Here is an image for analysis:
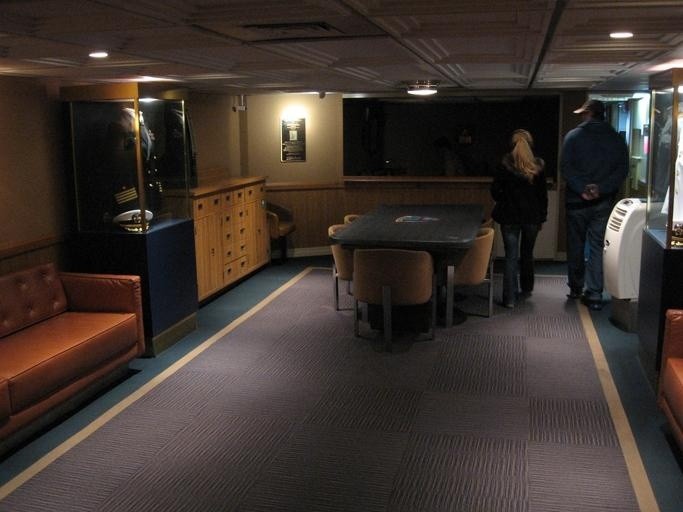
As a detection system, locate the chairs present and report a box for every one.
[265,196,296,264]
[324,200,499,354]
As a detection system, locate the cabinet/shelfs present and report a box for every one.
[156,171,274,309]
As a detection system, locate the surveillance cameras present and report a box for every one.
[232,105,245,112]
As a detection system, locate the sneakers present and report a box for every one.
[505,304,515,308]
[569,286,583,299]
[580,294,603,310]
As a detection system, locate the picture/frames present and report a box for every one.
[278,115,310,164]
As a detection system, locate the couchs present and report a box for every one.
[0,260,148,461]
[654,307,682,450]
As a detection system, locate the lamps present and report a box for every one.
[397,79,441,98]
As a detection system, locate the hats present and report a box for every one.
[572,97,606,116]
[110,209,154,233]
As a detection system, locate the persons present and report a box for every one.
[559,99,629,310]
[489,128,548,308]
